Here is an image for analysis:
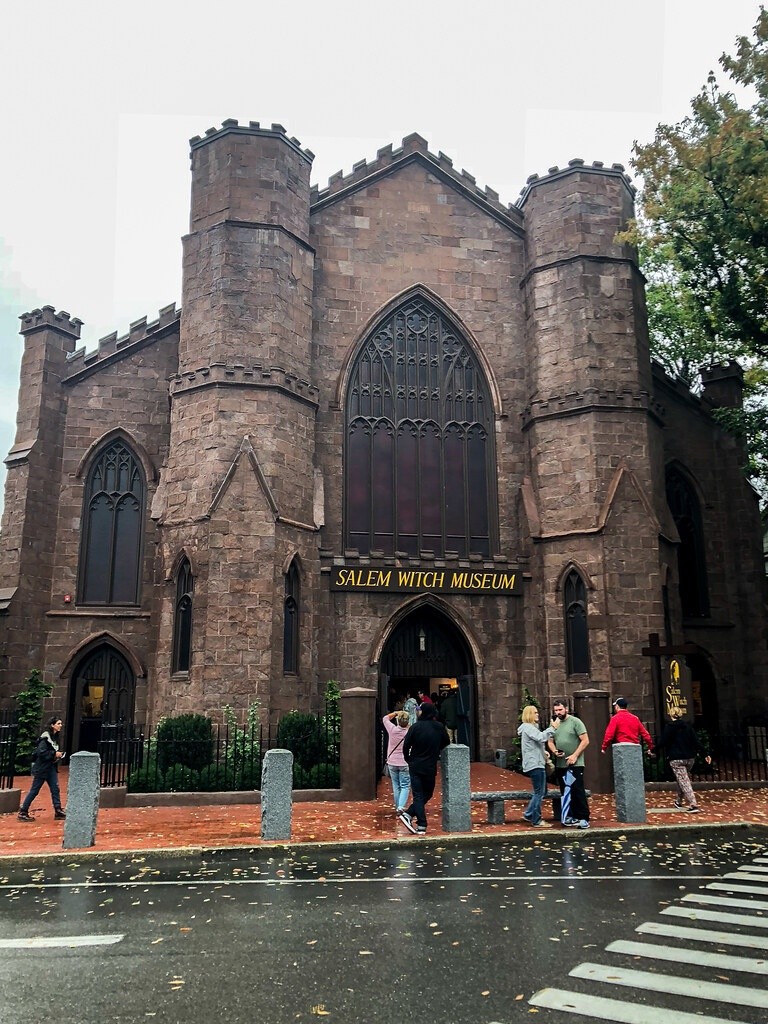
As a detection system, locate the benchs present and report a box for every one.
[471,788,591,825]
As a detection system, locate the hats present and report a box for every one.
[613,697,627,706]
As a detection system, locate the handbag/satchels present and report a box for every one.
[381,761,392,779]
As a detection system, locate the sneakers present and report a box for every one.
[399,812,417,835]
[397,809,403,816]
[415,825,427,836]
[533,820,555,828]
[686,807,700,814]
[522,814,533,822]
[674,801,682,808]
[55,812,66,820]
[16,814,35,822]
[561,817,573,827]
[575,819,591,829]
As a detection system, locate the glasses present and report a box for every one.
[535,711,538,714]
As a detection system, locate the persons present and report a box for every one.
[517,705,560,828]
[647,706,712,813]
[399,702,450,836]
[601,698,655,757]
[389,687,462,745]
[17,715,67,822]
[382,710,412,820]
[548,700,590,829]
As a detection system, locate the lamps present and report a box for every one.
[419,625,426,652]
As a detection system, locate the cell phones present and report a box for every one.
[552,715,558,721]
[558,749,564,755]
